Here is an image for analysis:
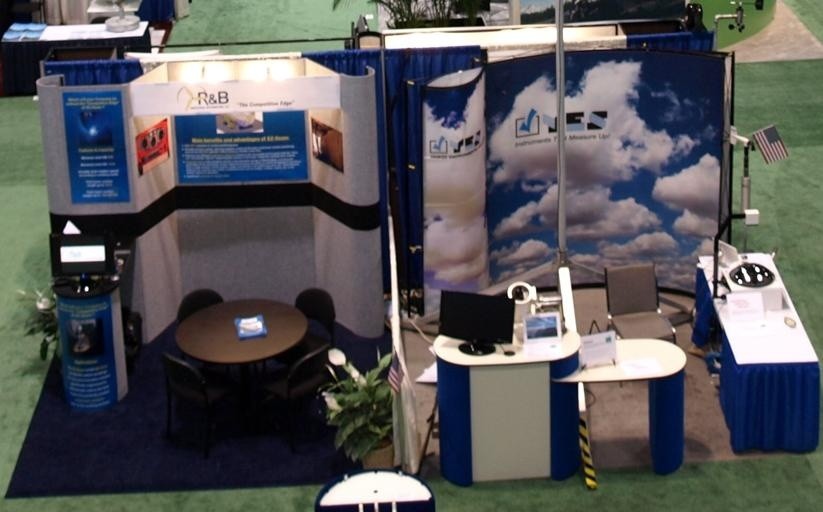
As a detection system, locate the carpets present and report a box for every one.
[402,282,772,471]
[5,331,393,500]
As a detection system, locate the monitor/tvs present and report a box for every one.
[49,233,114,293]
[438,290,516,357]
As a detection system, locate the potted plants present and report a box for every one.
[315,352,399,476]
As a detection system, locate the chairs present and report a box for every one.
[156,287,340,459]
[602,258,678,345]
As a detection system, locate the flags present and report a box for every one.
[385,352,407,394]
[751,125,790,165]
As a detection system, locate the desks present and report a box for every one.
[0,22,153,62]
[431,329,689,486]
[697,253,820,456]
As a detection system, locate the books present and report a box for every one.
[232,312,267,343]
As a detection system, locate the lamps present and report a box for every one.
[709,206,761,302]
